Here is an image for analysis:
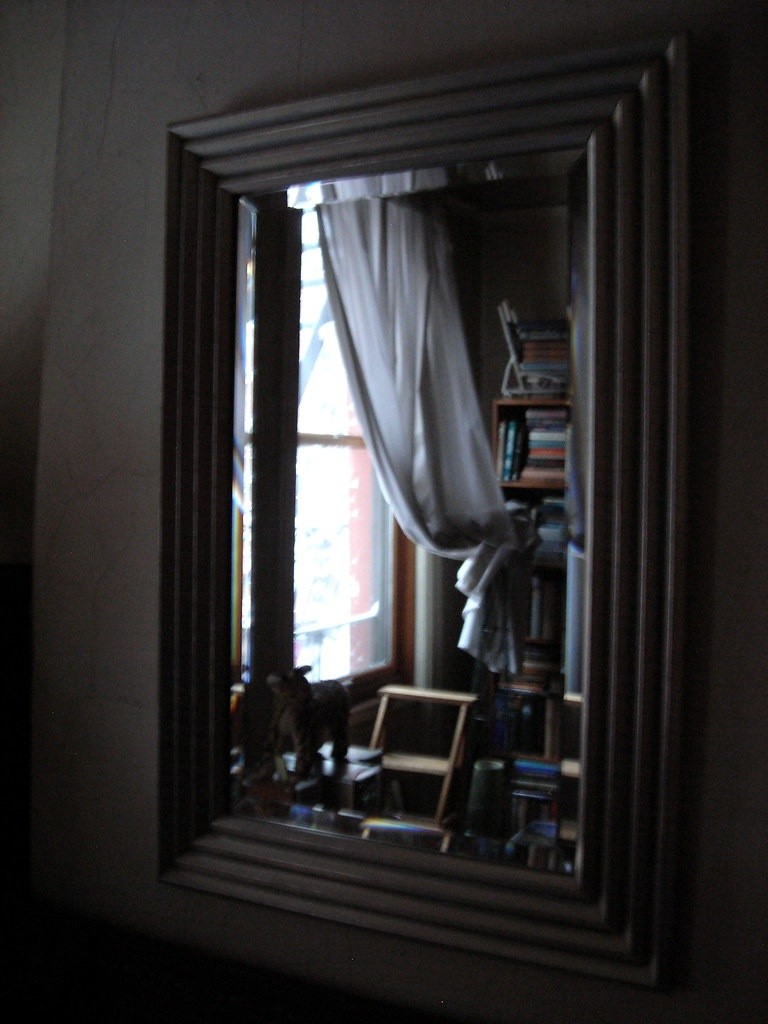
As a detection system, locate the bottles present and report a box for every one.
[290,804,336,832]
[527,566,563,645]
[522,638,560,687]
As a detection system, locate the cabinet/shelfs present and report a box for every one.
[473,304,581,763]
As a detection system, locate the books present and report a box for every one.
[518,319,569,371]
[491,647,550,750]
[528,575,555,641]
[495,409,568,483]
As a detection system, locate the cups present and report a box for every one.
[464,758,505,829]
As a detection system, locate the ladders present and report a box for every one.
[367,685,480,832]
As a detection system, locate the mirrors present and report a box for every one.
[152,35,730,998]
